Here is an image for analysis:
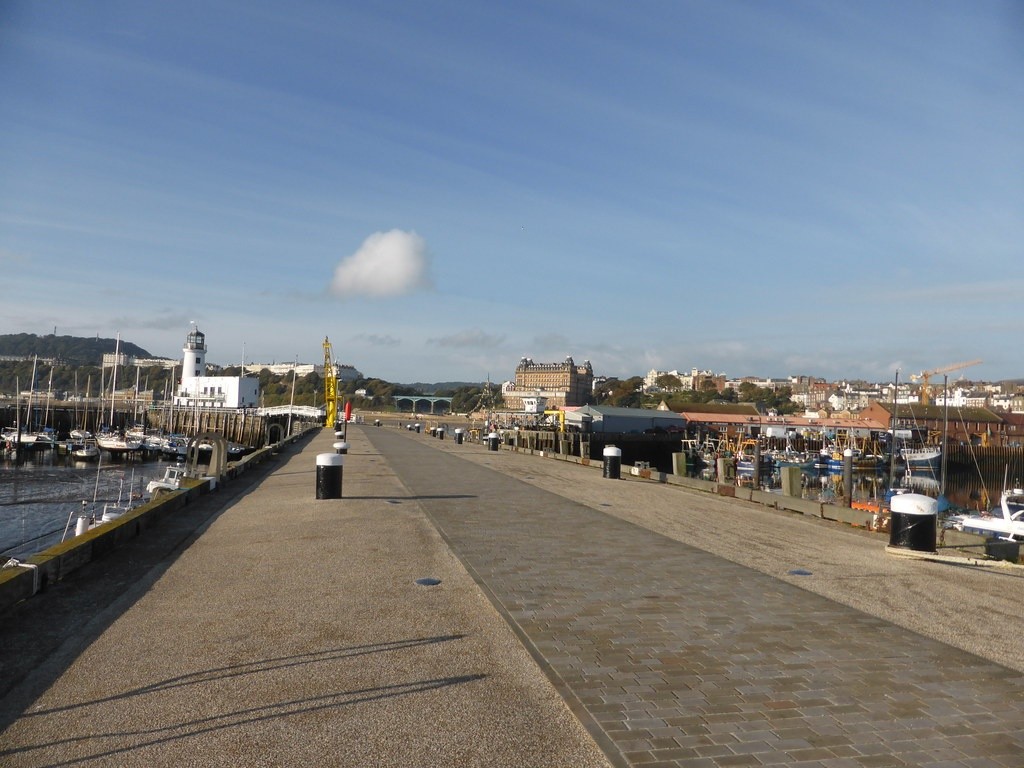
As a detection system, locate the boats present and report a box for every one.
[681,365,1024,544]
[0,331,257,533]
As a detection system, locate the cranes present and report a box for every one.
[910,360,982,406]
